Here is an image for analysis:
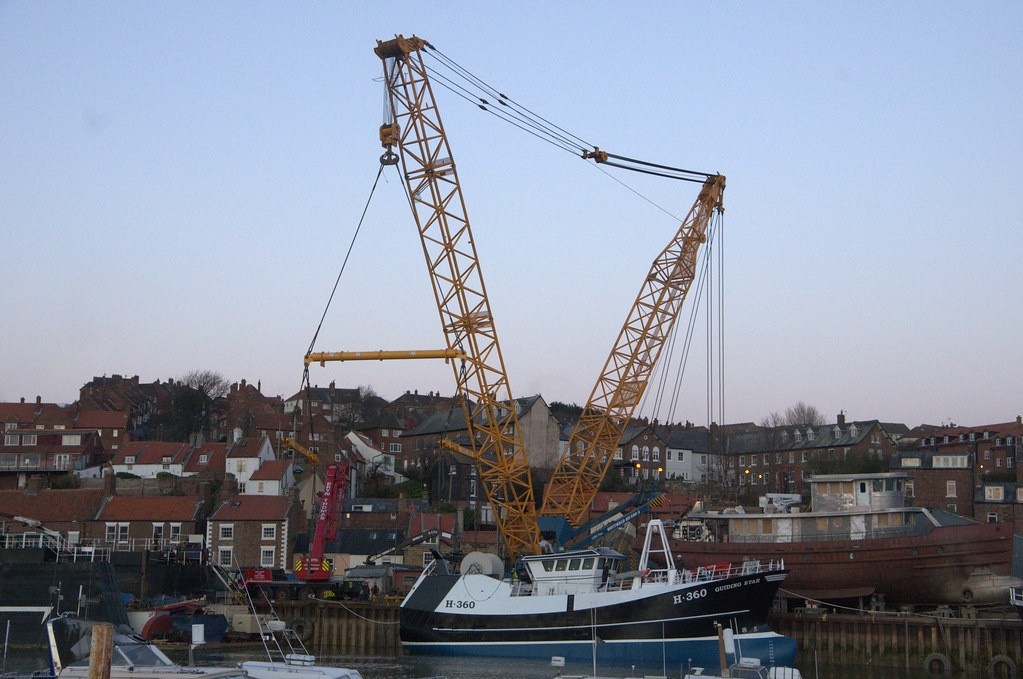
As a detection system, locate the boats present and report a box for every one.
[395,547,804,675]
[236,659,362,679]
[617,502,1016,608]
[28,637,248,679]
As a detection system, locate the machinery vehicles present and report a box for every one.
[294,460,347,581]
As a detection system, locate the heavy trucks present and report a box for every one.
[231,569,362,604]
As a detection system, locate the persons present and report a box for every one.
[153,530,159,551]
[675,555,684,571]
[597,563,609,592]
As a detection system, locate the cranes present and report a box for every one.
[282,35,726,563]
